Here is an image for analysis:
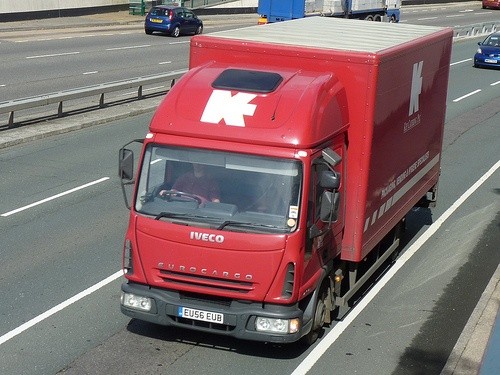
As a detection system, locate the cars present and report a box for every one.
[482,0,500,10]
[473,30,500,71]
[143,5,203,38]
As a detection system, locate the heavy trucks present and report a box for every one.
[256,0,403,27]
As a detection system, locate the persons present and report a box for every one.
[158,162,222,203]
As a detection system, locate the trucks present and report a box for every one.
[117,15,454,346]
